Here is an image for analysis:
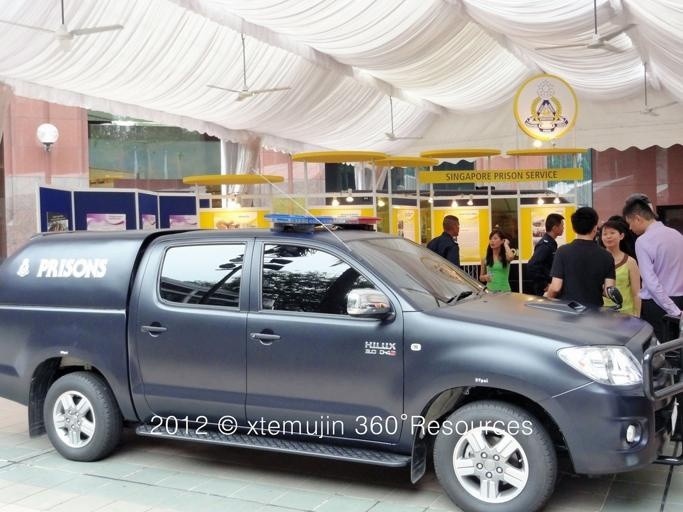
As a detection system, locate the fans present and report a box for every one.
[370,90,423,144]
[0,0,124,84]
[207,32,291,103]
[621,61,678,117]
[535,0,636,54]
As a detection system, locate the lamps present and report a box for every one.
[451,199,458,208]
[346,188,354,203]
[376,196,385,207]
[331,192,339,208]
[536,194,545,204]
[552,192,561,204]
[36,122,60,153]
[466,193,474,206]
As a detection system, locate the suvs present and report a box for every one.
[0,214,676,512]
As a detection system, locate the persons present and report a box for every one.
[525,194,683,443]
[479,230,516,293]
[425,214,460,268]
